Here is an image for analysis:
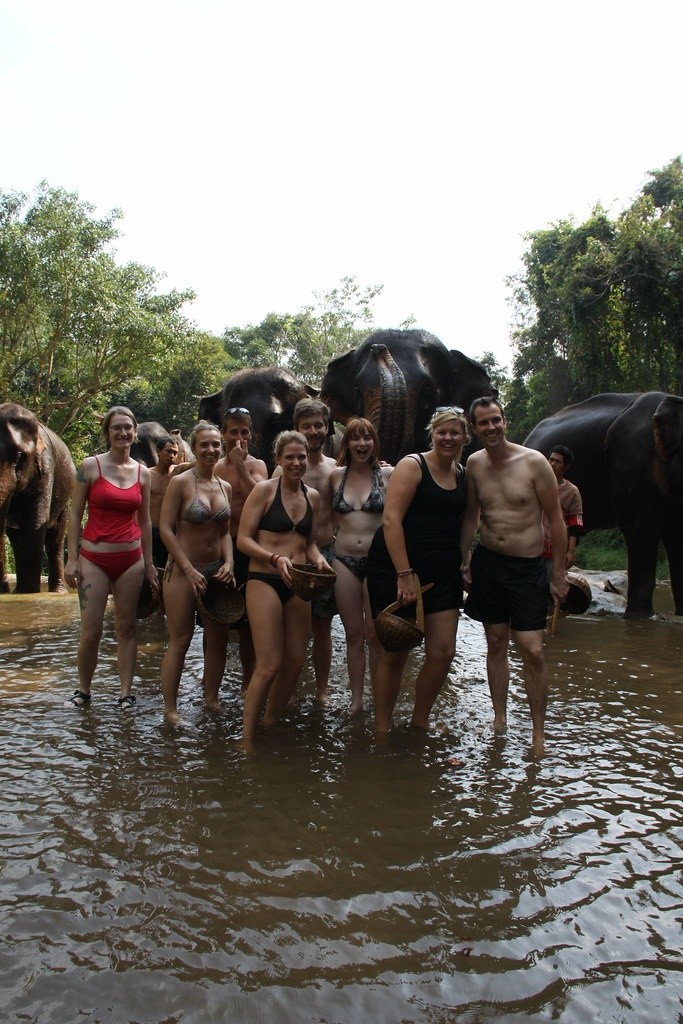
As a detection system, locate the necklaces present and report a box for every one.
[195,474,218,492]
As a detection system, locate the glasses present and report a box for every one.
[223,408,250,419]
[433,405,464,417]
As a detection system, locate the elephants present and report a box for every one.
[319,329,500,464]
[0,402,78,594]
[130,422,196,469]
[198,370,345,478]
[525,391,683,622]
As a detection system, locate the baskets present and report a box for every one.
[546,577,592,635]
[195,576,245,626]
[373,573,434,652]
[135,579,161,619]
[287,563,337,601]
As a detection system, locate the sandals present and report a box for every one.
[64,690,92,709]
[118,696,138,713]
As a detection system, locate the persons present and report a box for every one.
[541,445,583,635]
[160,420,236,718]
[460,396,571,757]
[272,398,392,700]
[236,430,336,738]
[64,406,160,710]
[148,436,178,619]
[366,406,466,733]
[196,407,268,698]
[329,418,395,710]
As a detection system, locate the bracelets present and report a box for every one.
[270,554,278,565]
[273,555,281,568]
[397,569,413,577]
